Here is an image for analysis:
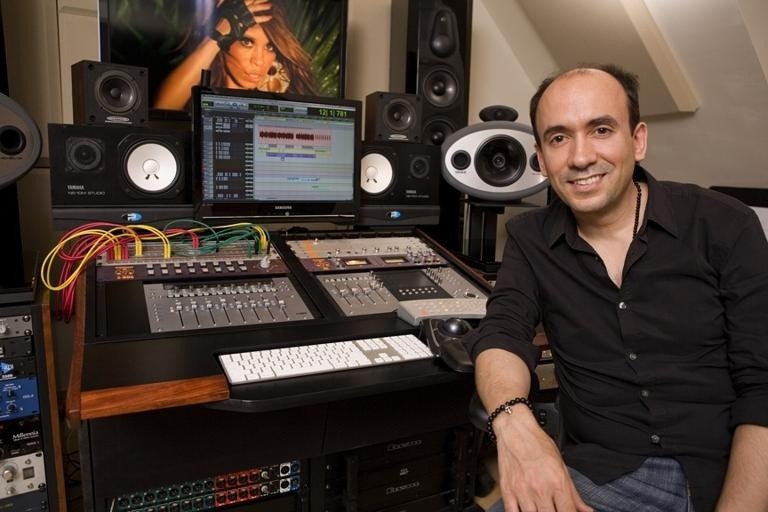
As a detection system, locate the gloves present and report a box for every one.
[210,0,257,51]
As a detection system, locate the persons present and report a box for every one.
[153,0,321,111]
[470,62,768,512]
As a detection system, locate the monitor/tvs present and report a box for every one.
[189,84,364,226]
[96,2,349,124]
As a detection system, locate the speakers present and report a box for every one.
[71,58,149,126]
[2,94,44,185]
[365,90,425,146]
[389,0,473,254]
[359,142,443,227]
[441,103,550,207]
[46,120,201,212]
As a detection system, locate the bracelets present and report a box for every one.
[485,397,534,442]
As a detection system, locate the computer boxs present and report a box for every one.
[0,247,70,512]
[341,434,474,511]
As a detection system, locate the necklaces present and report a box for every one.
[631,177,643,239]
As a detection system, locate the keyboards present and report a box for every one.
[215,333,434,387]
